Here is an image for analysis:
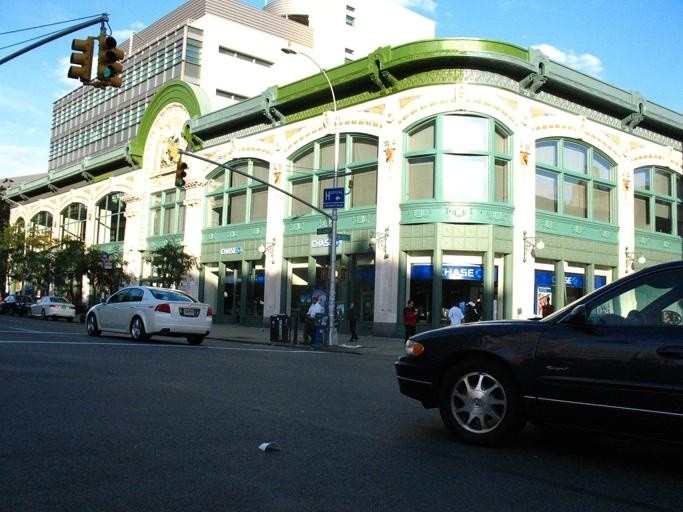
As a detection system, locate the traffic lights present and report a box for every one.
[111,47,125,88]
[175,161,187,187]
[67,38,94,82]
[100,36,117,81]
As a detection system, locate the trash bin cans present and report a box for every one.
[312,313,327,345]
[270,314,290,342]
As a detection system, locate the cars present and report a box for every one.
[86,285,214,346]
[0,295,35,317]
[27,295,77,322]
[392,259,683,448]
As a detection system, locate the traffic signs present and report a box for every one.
[281,46,367,351]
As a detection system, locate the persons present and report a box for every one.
[346,301,359,343]
[14,291,20,295]
[403,299,418,344]
[64,290,72,302]
[447,299,481,327]
[304,295,324,350]
